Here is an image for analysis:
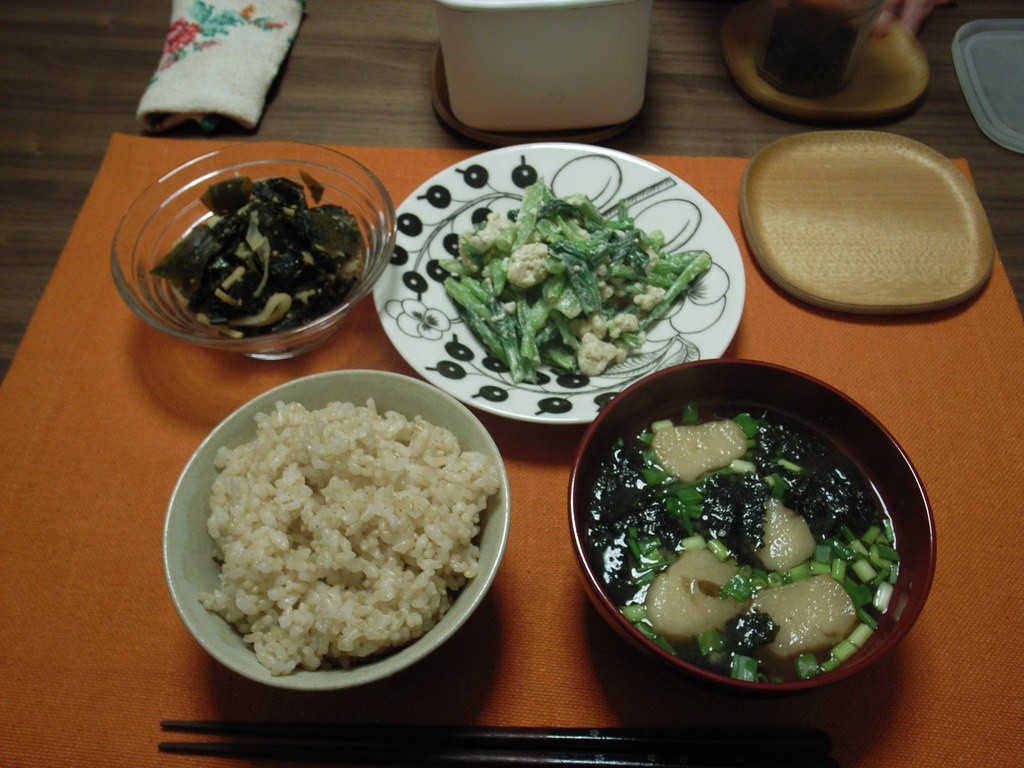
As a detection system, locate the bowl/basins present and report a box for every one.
[565,360,938,697]
[435,0,648,130]
[107,139,398,361]
[164,370,512,689]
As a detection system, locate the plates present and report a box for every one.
[372,142,746,424]
[431,42,638,145]
[717,0,928,121]
[737,131,995,314]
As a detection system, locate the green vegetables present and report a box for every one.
[442,181,710,382]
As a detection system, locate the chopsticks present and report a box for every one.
[158,719,836,768]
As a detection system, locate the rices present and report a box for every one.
[194,398,502,679]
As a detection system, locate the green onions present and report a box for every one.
[606,401,901,683]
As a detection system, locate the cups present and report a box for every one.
[753,0,885,99]
